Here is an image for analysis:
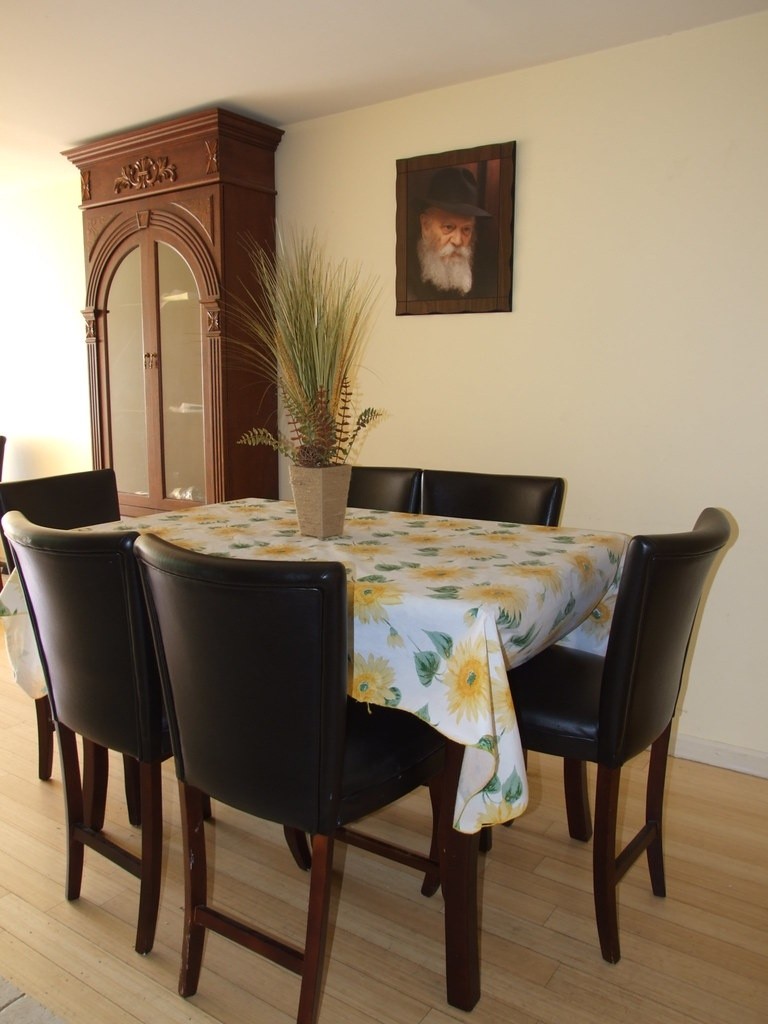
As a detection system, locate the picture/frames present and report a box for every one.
[392,136,518,316]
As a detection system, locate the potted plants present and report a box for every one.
[216,242,383,539]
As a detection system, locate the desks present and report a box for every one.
[0,497,633,1015]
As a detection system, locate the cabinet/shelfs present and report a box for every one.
[61,108,286,517]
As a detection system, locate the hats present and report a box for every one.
[412,167,493,217]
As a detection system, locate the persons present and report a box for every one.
[406,166,497,300]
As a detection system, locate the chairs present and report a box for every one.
[418,508,734,965]
[0,508,176,952]
[421,468,567,527]
[1,470,122,782]
[348,465,417,514]
[135,533,482,1024]
